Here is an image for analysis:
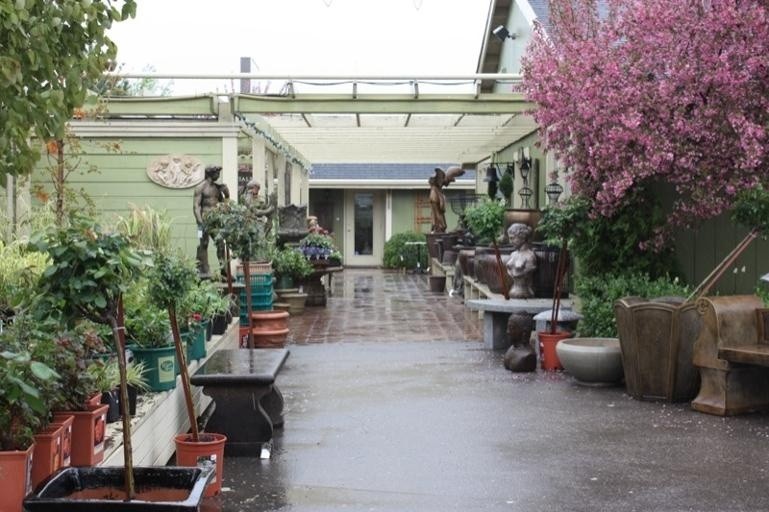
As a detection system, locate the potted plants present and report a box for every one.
[268,246,315,287]
[536,196,589,375]
[0,193,233,512]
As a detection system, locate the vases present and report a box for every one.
[475,243,515,294]
[423,233,475,292]
[557,333,627,389]
[246,289,308,347]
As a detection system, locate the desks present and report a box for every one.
[190,347,290,457]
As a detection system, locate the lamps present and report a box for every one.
[483,162,503,199]
[519,158,533,180]
[492,24,516,41]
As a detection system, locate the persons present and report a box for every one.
[505,221,537,298]
[243,178,274,240]
[192,164,238,285]
